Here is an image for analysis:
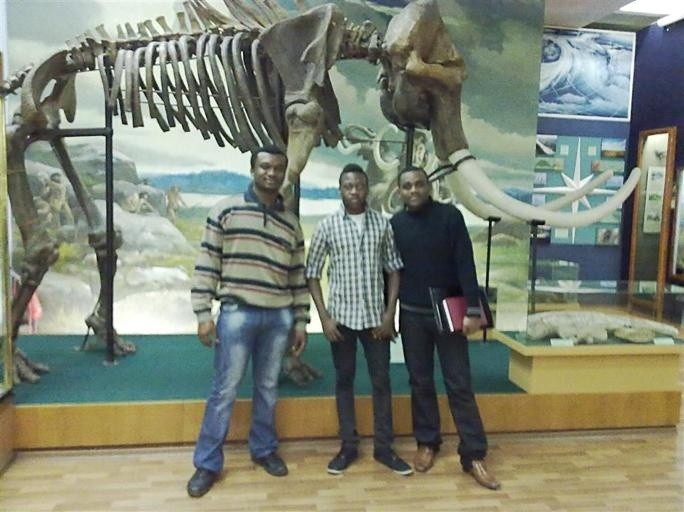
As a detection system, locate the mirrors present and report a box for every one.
[629,124,676,316]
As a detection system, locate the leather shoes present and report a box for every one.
[413,445,437,472]
[186,467,216,498]
[461,459,501,490]
[250,450,289,476]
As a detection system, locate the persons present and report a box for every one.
[539,31,632,105]
[185,144,311,498]
[381,167,503,491]
[304,162,417,480]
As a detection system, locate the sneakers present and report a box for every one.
[327,445,361,475]
[373,445,414,476]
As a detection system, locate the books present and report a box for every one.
[426,283,494,334]
[435,302,449,334]
[443,294,489,334]
